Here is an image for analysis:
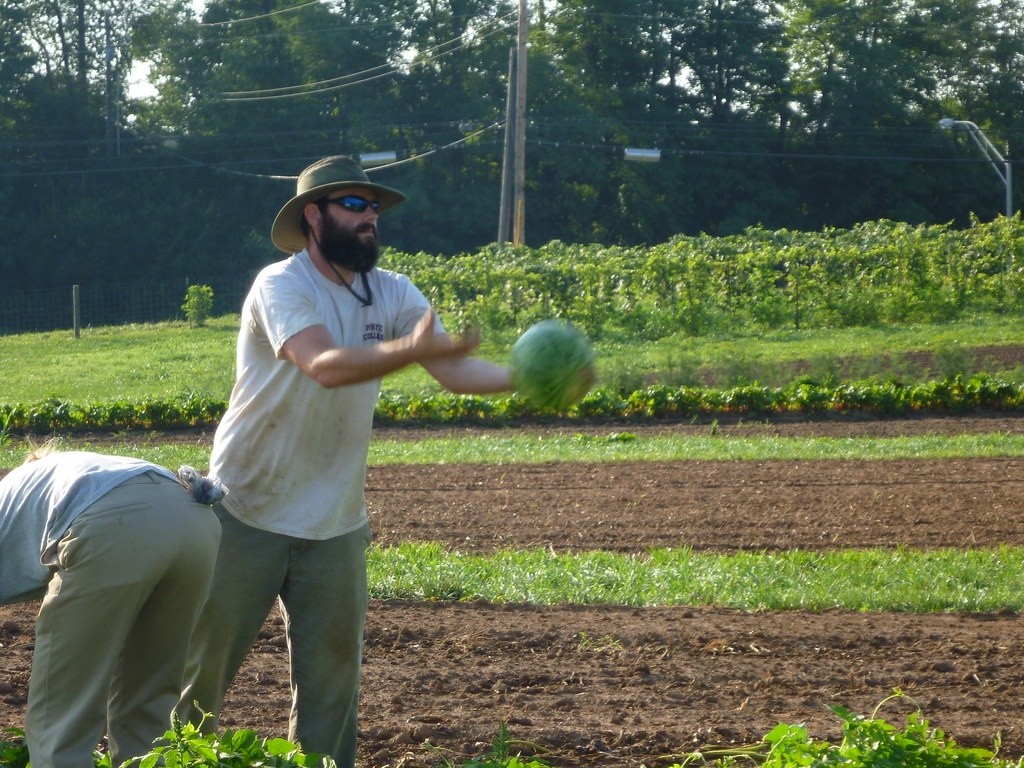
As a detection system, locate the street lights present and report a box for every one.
[939,116,1012,218]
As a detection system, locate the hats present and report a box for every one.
[273,154,407,254]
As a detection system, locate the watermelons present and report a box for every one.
[509,319,595,410]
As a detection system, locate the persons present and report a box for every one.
[210,156,519,768]
[0,450,223,768]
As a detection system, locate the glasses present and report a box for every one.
[325,196,382,215]
